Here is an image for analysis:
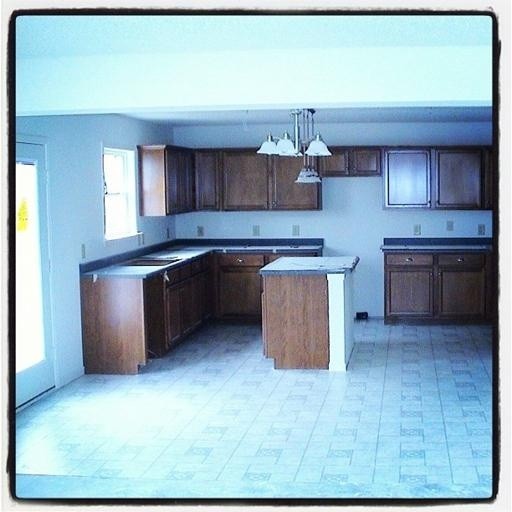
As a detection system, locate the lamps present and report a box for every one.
[256,108,332,184]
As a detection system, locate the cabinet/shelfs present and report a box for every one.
[320,144,383,176]
[383,144,492,209]
[137,144,194,217]
[160,252,214,356]
[383,249,487,325]
[193,148,221,210]
[222,147,321,211]
[266,252,317,265]
[215,254,265,320]
